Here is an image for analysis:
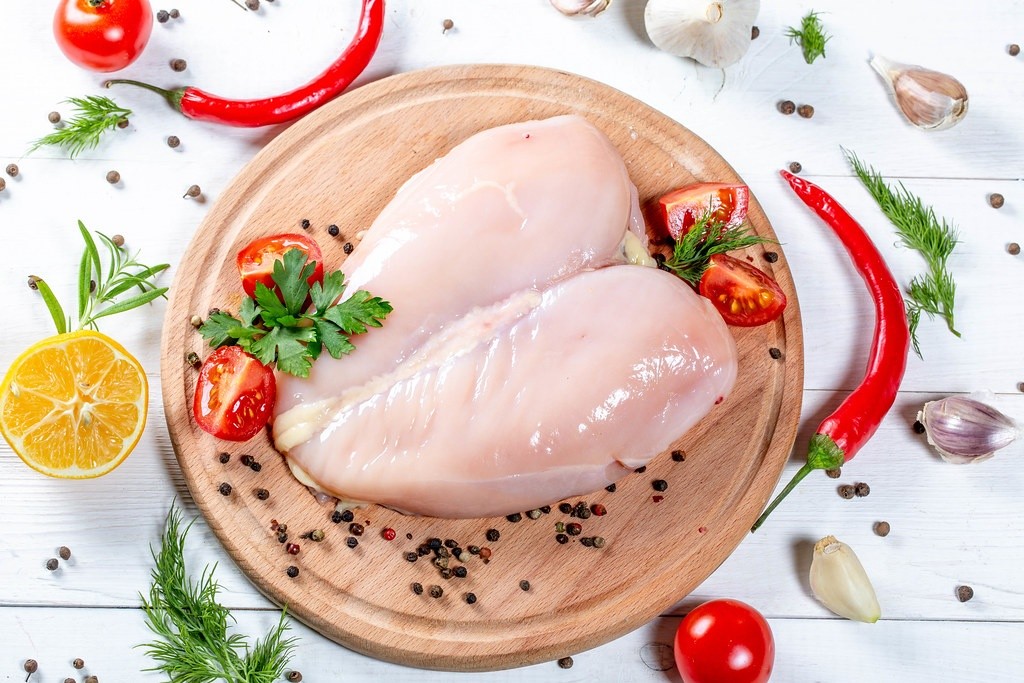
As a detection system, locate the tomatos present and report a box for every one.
[673,599,775,683]
[55,0,155,70]
[194,233,322,440]
[660,184,785,327]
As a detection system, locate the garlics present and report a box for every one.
[553,0,760,64]
[869,55,968,133]
[809,536,881,623]
[918,397,1020,460]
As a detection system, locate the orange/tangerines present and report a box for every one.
[0,331,147,477]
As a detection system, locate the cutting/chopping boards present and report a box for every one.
[160,62,806,671]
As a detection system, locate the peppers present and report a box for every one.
[106,1,386,128]
[751,170,909,538]
